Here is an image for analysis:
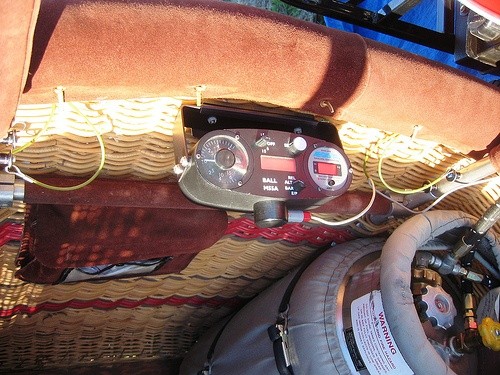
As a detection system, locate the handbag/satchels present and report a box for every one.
[15,203,228,285]
